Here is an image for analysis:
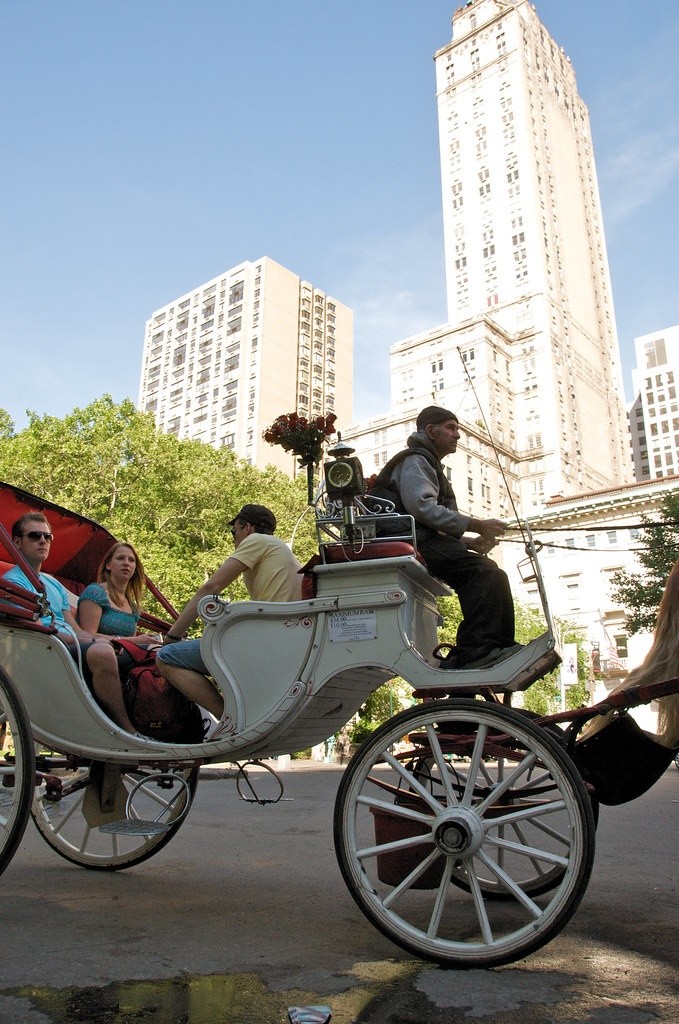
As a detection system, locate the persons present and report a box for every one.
[76,541,164,644]
[0,509,163,743]
[156,503,304,720]
[364,405,515,669]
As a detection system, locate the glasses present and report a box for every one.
[231,522,253,539]
[22,531,54,542]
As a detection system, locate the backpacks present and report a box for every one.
[111,638,212,743]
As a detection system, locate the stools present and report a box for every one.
[321,542,452,599]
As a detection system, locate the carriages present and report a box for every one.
[0,483,679,969]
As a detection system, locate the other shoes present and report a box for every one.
[440,644,522,670]
[134,731,165,743]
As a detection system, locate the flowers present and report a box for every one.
[262,412,338,503]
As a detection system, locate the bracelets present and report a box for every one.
[166,633,182,641]
[93,637,95,643]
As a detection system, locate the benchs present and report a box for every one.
[0,561,88,647]
[199,555,315,736]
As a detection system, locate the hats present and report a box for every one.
[417,406,459,432]
[226,504,276,532]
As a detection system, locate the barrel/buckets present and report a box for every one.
[368,792,461,890]
